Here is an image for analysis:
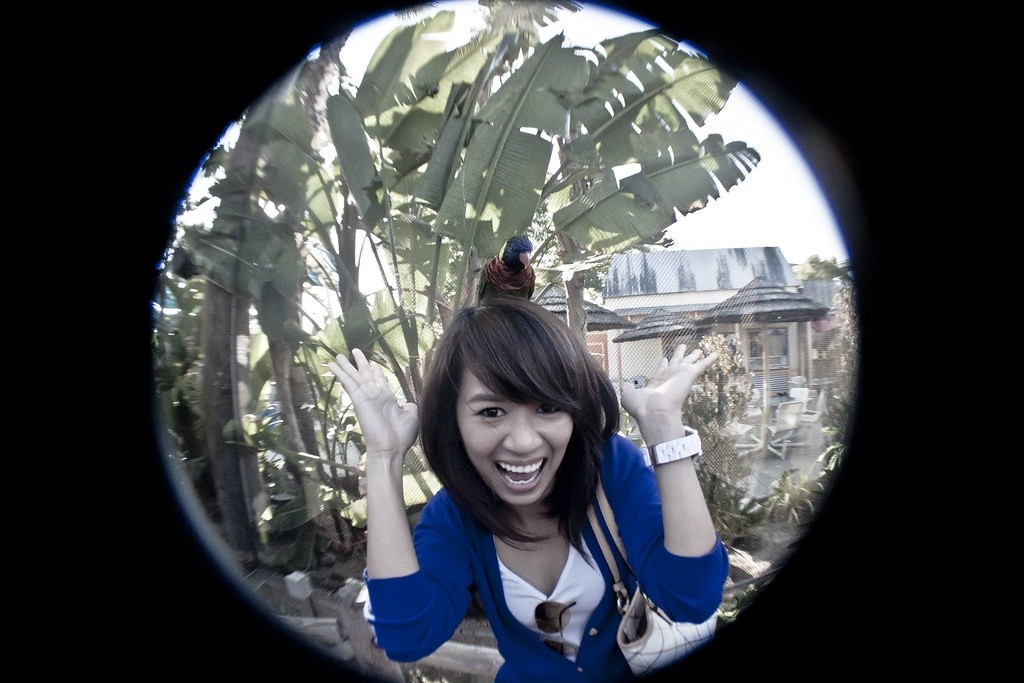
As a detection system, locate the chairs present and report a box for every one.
[718,375,827,460]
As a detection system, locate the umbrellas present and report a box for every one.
[526,275,830,459]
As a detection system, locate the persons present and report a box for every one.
[325,293,729,683]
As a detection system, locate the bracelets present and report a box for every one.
[647,424,705,466]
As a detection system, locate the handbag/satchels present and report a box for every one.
[586,475,726,676]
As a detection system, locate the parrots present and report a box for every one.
[475,235,537,307]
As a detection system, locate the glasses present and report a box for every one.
[534,600,578,658]
